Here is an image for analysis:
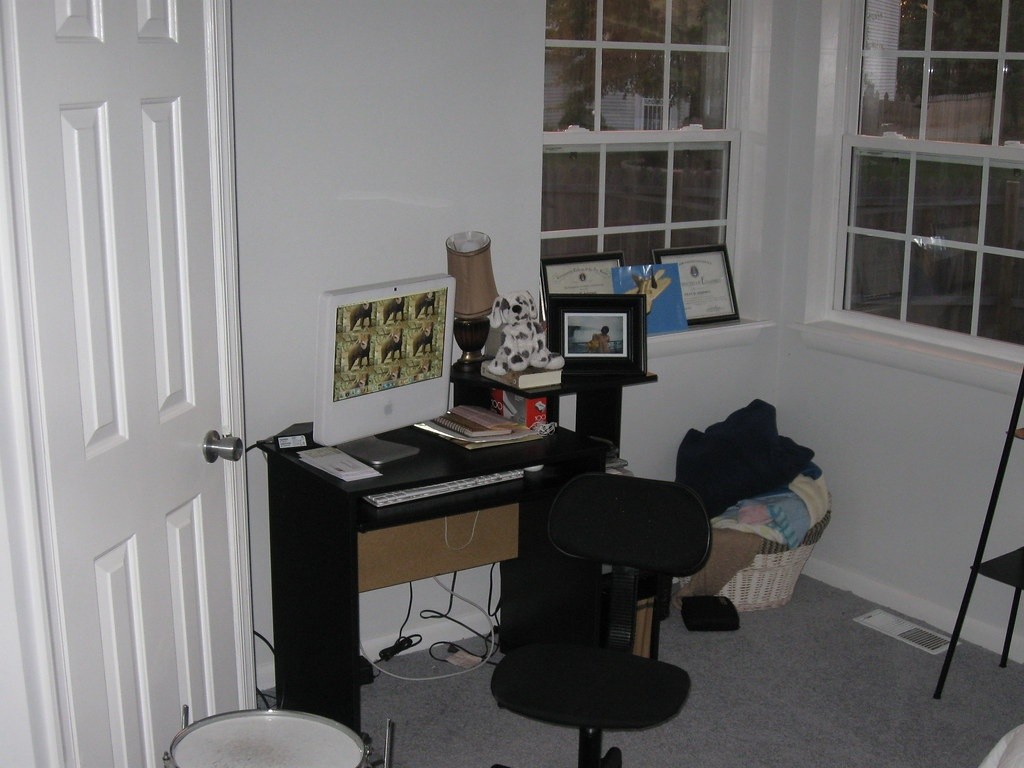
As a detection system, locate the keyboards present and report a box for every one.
[363,470,524,507]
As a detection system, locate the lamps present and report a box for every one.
[445,230,499,374]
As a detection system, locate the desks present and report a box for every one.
[450,356,657,457]
[254,427,609,747]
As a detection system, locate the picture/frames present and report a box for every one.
[652,244,741,328]
[540,250,626,316]
[546,293,648,377]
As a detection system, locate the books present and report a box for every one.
[414,405,543,451]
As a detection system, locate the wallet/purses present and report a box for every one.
[681,595,740,631]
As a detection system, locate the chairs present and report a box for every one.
[491,471,713,768]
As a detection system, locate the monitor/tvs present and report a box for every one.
[313,273,456,464]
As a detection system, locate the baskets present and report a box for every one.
[673,490,833,613]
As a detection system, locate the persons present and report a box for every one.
[587,326,611,353]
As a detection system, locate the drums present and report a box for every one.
[163,705,368,768]
